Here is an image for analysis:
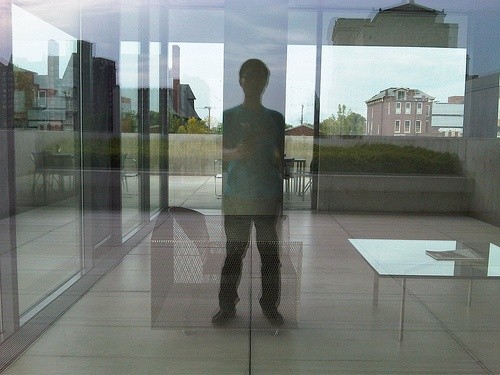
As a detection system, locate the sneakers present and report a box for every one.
[212,299,237,323]
[258,297,284,326]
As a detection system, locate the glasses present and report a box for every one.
[242,74,267,82]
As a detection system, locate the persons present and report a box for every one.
[209,58,285,328]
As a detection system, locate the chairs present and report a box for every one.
[300,160,311,198]
[123,154,128,192]
[42,155,74,199]
[29,152,59,194]
[284,158,293,201]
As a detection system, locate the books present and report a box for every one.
[426,247,484,261]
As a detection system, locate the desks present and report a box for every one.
[295,159,306,201]
[348,239,500,343]
